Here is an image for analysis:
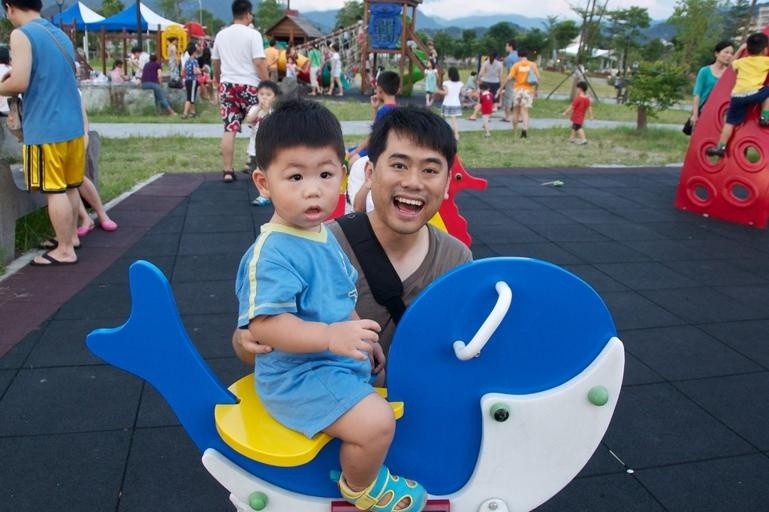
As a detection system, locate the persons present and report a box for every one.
[707,33,769,155]
[69,62,121,236]
[345,70,412,174]
[111,37,214,118]
[244,81,290,209]
[265,38,344,97]
[690,41,734,132]
[232,99,429,511]
[339,14,366,50]
[562,82,594,145]
[440,39,541,140]
[0,0,117,266]
[0,47,24,118]
[423,58,439,109]
[233,106,475,368]
[209,0,272,185]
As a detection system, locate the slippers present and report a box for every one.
[100,218,118,232]
[30,251,80,267]
[76,223,97,237]
[38,236,83,250]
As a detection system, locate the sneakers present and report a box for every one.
[705,146,726,158]
[337,464,428,512]
[758,116,769,129]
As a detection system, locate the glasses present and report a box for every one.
[249,10,253,17]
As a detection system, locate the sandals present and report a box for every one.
[222,168,237,183]
[250,195,272,207]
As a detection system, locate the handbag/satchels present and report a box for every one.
[6,95,24,144]
[317,65,322,77]
[527,68,540,85]
[682,115,692,136]
